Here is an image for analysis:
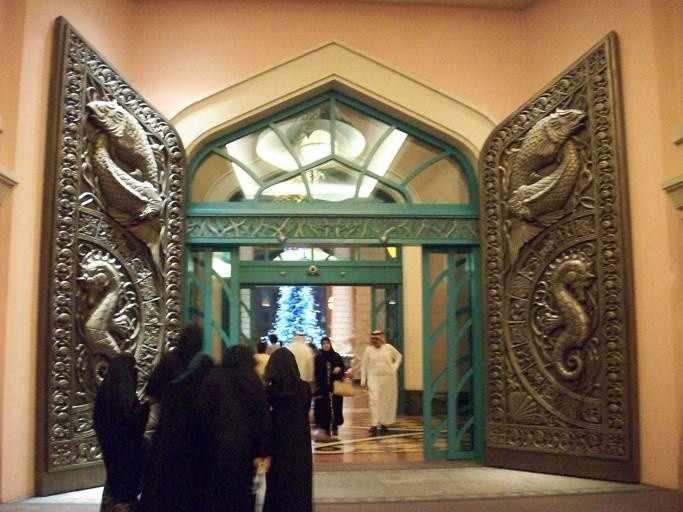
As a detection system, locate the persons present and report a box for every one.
[360,330,402,434]
[313,336,345,437]
[91,321,321,512]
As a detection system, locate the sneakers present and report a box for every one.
[369,425,387,434]
[324,425,338,436]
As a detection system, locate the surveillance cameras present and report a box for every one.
[309,266,317,273]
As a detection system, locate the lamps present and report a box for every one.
[255,118,366,173]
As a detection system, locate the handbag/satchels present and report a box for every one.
[334,380,355,397]
